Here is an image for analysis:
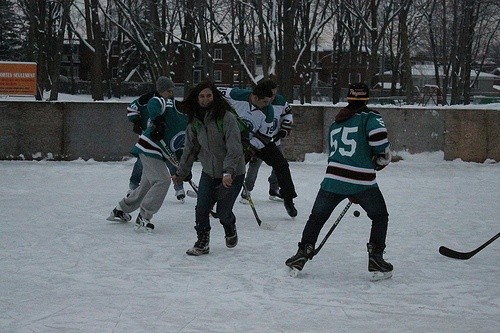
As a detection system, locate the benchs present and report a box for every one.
[460,92,500,105]
[369,96,408,105]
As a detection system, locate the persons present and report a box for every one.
[167,81,246,256]
[106,97,201,233]
[216,85,298,220]
[228,80,292,205]
[285,82,394,282]
[126,76,186,204]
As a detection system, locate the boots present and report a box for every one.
[223,224,238,248]
[367,242,393,280]
[285,242,311,277]
[186,226,210,255]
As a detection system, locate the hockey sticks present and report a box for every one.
[242,183,278,231]
[439,232,500,260]
[159,139,221,219]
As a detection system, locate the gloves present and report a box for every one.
[272,130,286,142]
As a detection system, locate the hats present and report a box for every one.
[347,82,370,100]
[156,76,174,92]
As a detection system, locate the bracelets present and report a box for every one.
[223,173,232,176]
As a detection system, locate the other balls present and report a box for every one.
[354,211,360,218]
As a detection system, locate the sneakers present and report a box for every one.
[135,213,154,230]
[241,187,251,200]
[107,208,131,222]
[176,188,185,201]
[284,198,297,217]
[269,190,283,201]
